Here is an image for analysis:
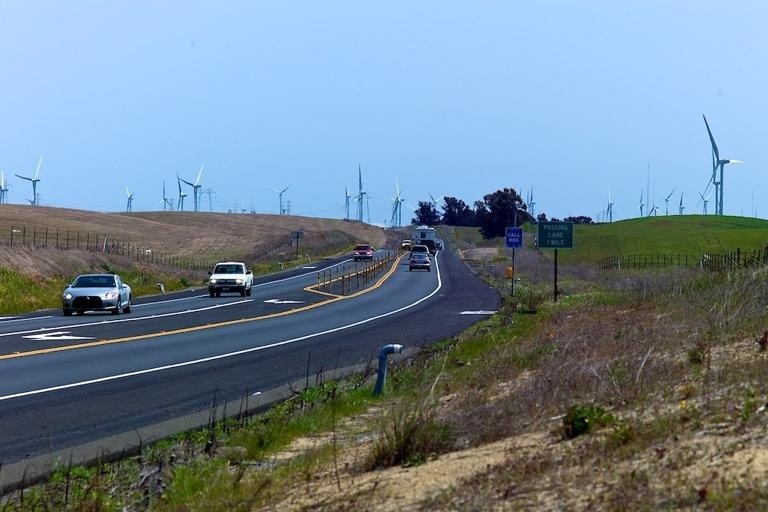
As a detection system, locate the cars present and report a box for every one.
[401,225,445,272]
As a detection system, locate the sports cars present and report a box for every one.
[64,271,130,316]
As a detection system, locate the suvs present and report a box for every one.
[353,243,374,262]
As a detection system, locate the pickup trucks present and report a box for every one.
[208,261,254,295]
